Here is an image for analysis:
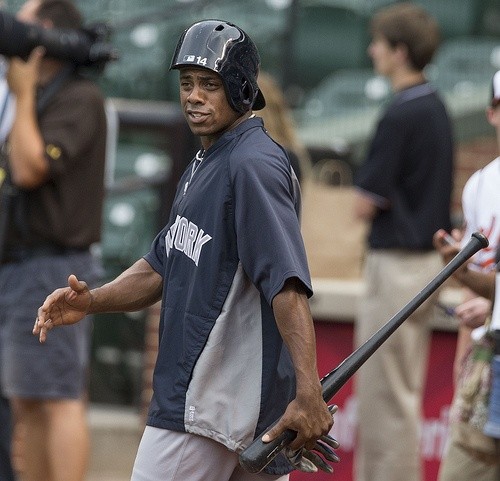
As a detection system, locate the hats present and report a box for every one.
[491,70,500,107]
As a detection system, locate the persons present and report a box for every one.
[255,74,303,176]
[0,0,106,481]
[434,69,500,481]
[33,20,333,481]
[353,7,453,481]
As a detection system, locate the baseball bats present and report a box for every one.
[238,231,489,475]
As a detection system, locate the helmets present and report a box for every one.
[167,18,265,113]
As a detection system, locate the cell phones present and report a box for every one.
[442,233,464,252]
